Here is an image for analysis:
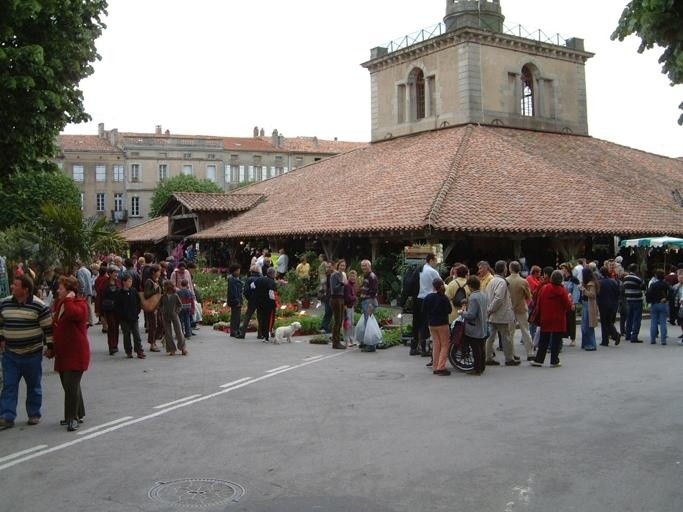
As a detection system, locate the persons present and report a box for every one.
[0,274,54,430]
[49,275,89,432]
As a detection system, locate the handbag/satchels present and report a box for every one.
[135,278,163,314]
[527,303,541,326]
[101,299,117,311]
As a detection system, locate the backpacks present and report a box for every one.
[451,278,470,308]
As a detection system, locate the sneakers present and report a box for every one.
[0,418,16,428]
[28,416,40,425]
[409,318,682,377]
[229,328,275,343]
[86,320,200,360]
[329,336,377,353]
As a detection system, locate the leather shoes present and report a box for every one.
[60,418,85,432]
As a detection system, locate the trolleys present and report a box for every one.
[400,311,413,346]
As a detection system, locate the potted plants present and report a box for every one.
[191,248,412,348]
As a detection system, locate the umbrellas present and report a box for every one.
[617,235,682,277]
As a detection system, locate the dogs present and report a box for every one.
[274,322,302,344]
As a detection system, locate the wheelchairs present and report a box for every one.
[448,336,479,372]
